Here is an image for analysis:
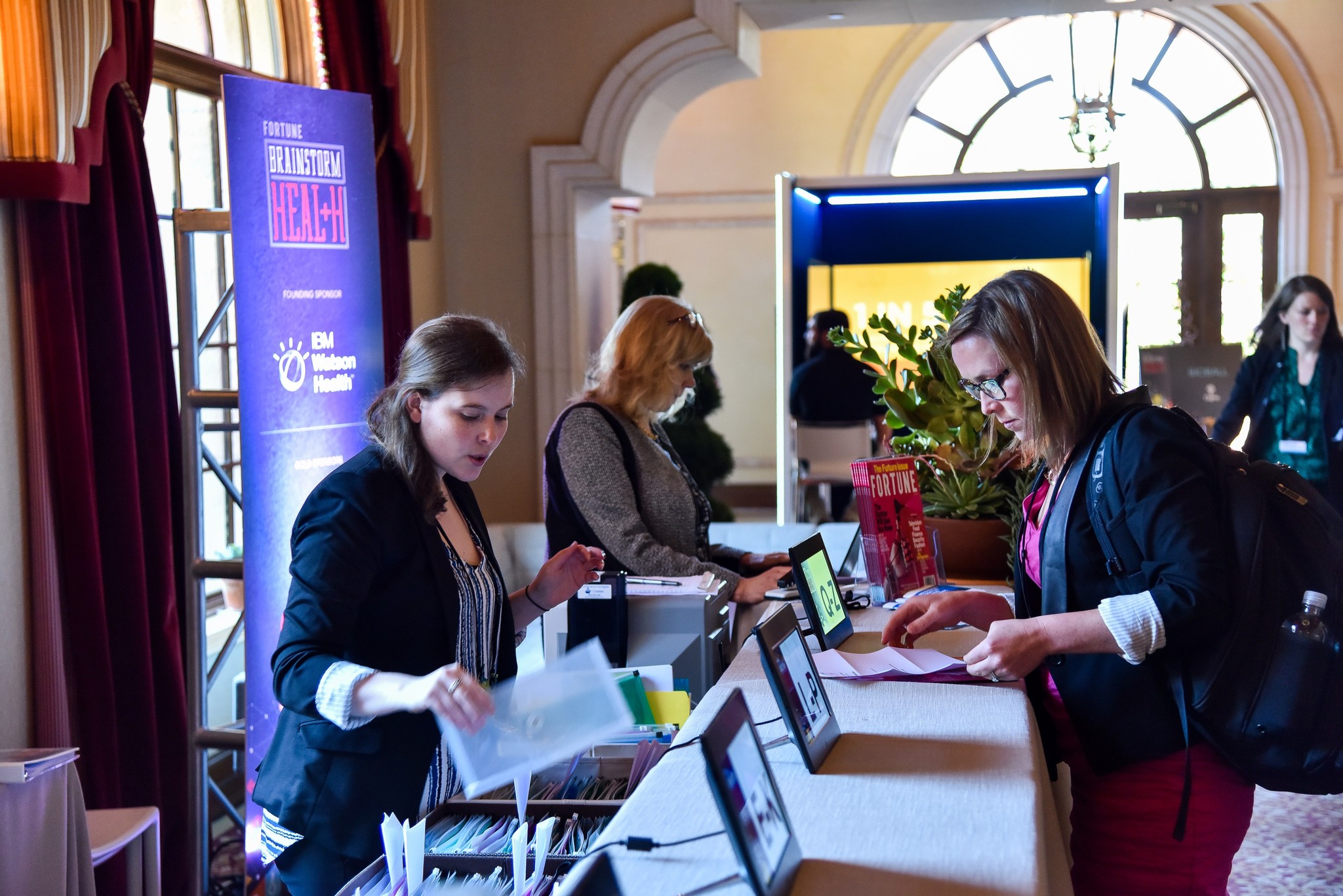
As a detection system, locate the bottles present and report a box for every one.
[1283,589,1331,642]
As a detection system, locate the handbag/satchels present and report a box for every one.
[1085,404,1343,796]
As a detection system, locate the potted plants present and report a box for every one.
[823,281,1036,581]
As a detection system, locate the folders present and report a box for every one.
[0,747,81,784]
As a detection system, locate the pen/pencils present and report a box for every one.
[626,578,685,586]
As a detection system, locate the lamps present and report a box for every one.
[1057,10,1128,163]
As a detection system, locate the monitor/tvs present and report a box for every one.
[788,532,854,651]
[700,688,801,896]
[756,602,840,776]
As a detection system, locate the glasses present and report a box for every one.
[665,312,703,328]
[958,363,1013,403]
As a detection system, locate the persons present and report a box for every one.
[1208,275,1343,504]
[541,293,795,658]
[788,308,892,523]
[880,269,1257,896]
[251,314,606,896]
[621,263,738,524]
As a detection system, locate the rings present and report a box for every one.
[989,672,1000,682]
[448,677,464,695]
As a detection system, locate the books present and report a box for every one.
[849,457,938,607]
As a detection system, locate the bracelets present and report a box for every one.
[525,584,550,612]
